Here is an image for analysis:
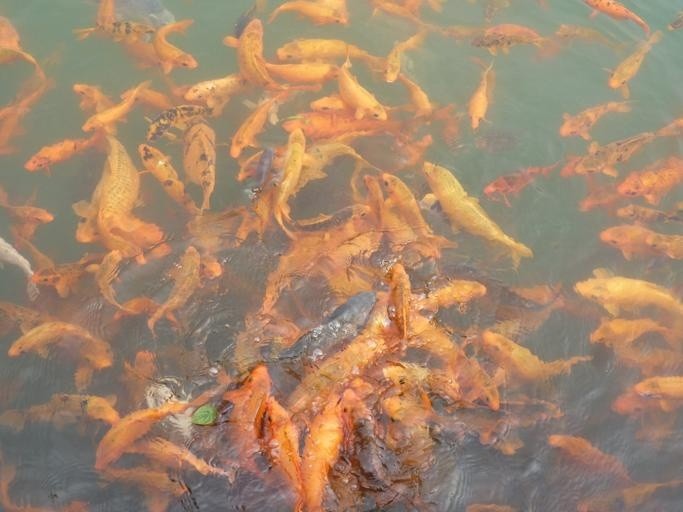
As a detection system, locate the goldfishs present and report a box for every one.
[0,1,681,512]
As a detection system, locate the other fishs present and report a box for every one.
[0,1,681,512]
